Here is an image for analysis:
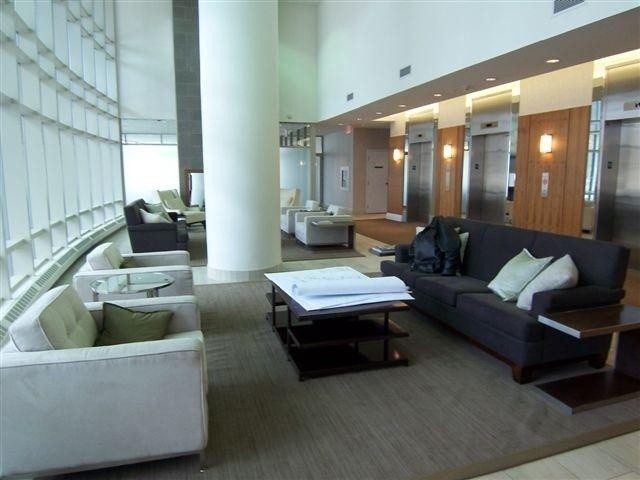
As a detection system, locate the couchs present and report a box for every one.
[125,198,187,253]
[379,215,630,385]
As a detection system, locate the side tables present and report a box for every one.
[534,303,639,414]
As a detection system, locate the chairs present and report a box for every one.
[279,187,356,249]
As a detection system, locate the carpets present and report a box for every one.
[352,218,429,247]
[33,271,639,480]
[179,221,367,266]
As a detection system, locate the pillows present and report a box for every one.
[415,226,469,278]
[140,202,173,224]
[486,247,580,311]
[164,196,186,212]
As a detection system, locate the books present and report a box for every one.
[371,245,396,253]
